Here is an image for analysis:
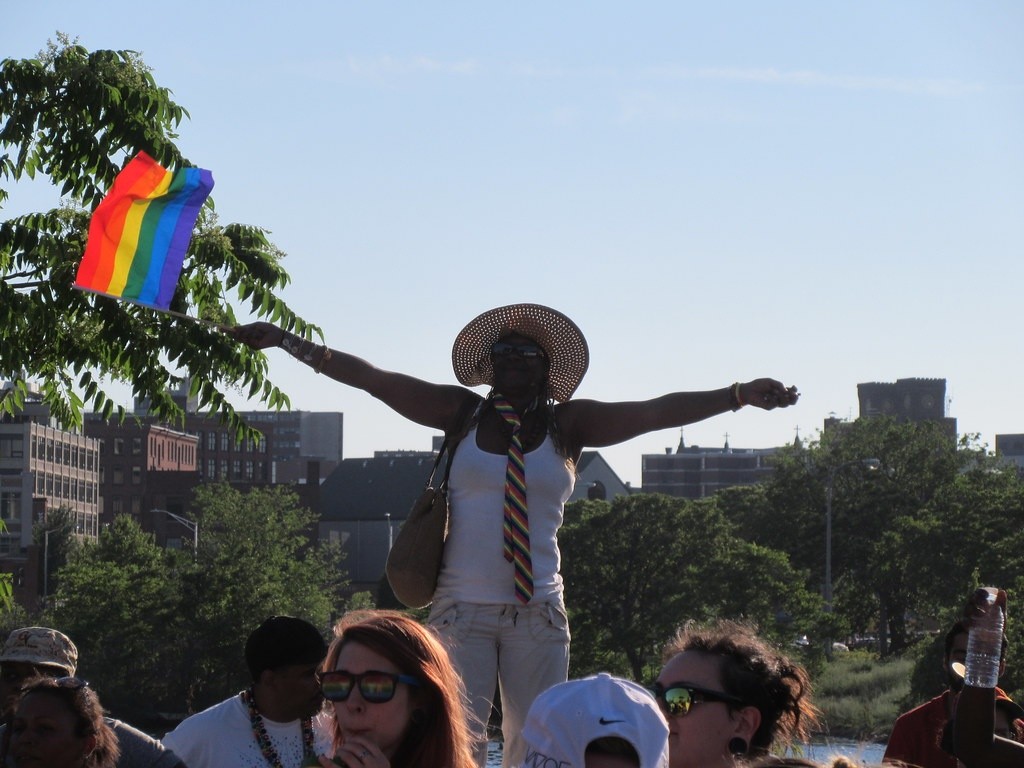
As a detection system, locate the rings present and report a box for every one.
[358,749,370,761]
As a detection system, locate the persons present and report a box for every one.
[1,585,1024,768]
[221,305,799,768]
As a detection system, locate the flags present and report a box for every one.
[74,150,215,312]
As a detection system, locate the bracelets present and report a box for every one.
[277,329,332,373]
[728,382,745,412]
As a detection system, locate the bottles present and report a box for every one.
[964,587,1005,689]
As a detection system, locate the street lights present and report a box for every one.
[825,460,880,658]
[151,510,199,563]
[44,529,61,595]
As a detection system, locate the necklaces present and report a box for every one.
[495,410,542,452]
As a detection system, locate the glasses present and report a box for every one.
[21,677,88,691]
[493,342,544,358]
[320,671,416,703]
[646,685,729,716]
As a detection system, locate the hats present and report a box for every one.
[521,672,670,768]
[452,303,589,404]
[245,615,327,672]
[0,627,78,678]
[995,687,1024,716]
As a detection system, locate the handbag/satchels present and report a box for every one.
[386,397,483,610]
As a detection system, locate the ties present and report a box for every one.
[491,390,542,604]
